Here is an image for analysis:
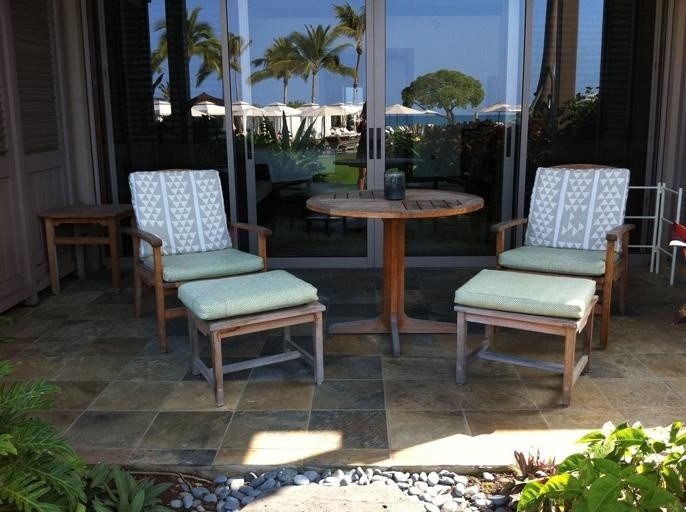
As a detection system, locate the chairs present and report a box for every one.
[491,163,636,348]
[130,168,273,354]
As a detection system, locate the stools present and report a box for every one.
[178,269,325,406]
[453,269,599,407]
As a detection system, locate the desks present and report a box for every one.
[306,188,484,358]
[39,204,132,295]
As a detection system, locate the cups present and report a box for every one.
[383,170,405,201]
